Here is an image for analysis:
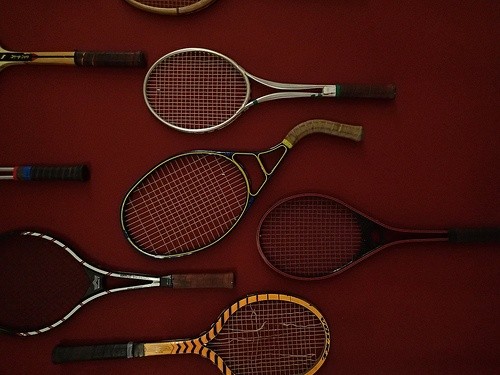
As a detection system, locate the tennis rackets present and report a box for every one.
[122,119,363,261]
[255,193,499,282]
[144,49,394,133]
[129,0,208,14]
[0,165,90,183]
[50,294,329,375]
[0,230,235,336]
[0,46,145,69]
